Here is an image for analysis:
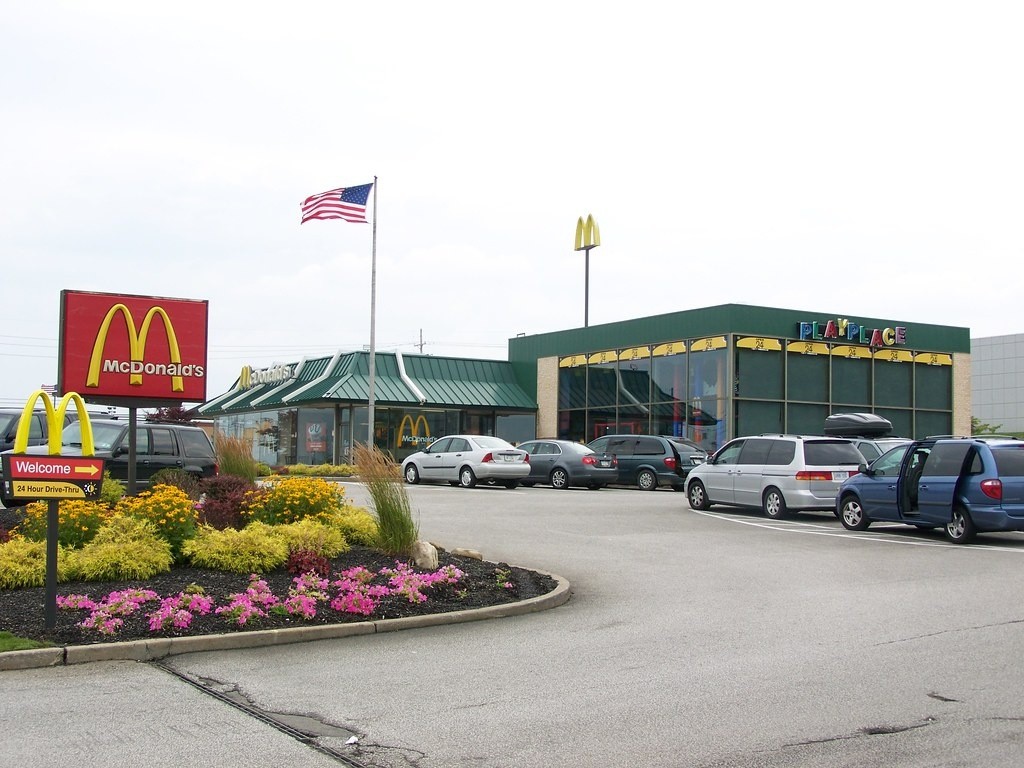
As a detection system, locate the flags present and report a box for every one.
[41,384,57,396]
[298,182,374,227]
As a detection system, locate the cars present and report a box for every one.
[487,439,619,490]
[401,435,531,489]
[586,434,709,491]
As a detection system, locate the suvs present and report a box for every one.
[684,433,870,520]
[823,412,941,471]
[0,416,219,509]
[836,434,1024,544]
[0,407,111,453]
